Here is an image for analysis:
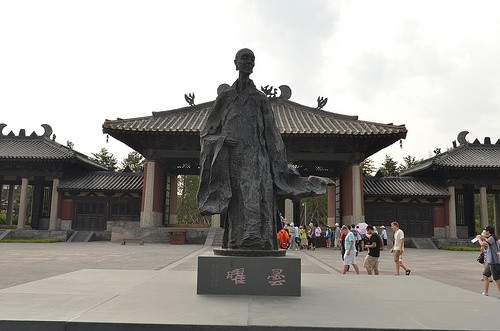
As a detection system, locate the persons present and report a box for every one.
[277,213,388,271]
[195,48,335,249]
[476,224,500,296]
[364,225,381,275]
[341,226,359,275]
[390,221,411,275]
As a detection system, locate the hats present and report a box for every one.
[380,226,386,228]
[483,225,494,234]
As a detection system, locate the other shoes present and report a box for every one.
[482,293,489,296]
[406,269,411,275]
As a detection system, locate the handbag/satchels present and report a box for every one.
[477,253,485,264]
